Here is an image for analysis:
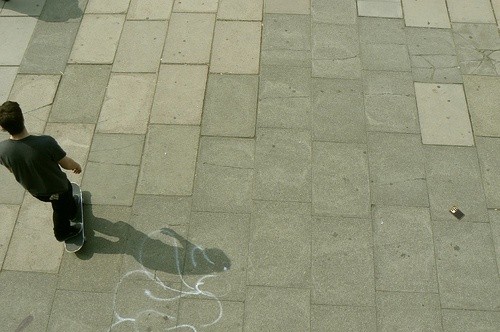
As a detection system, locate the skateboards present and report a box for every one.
[63,182,86,253]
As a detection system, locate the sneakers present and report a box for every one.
[71,194,80,221]
[59,223,82,242]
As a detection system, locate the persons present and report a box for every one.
[0,101,83,242]
